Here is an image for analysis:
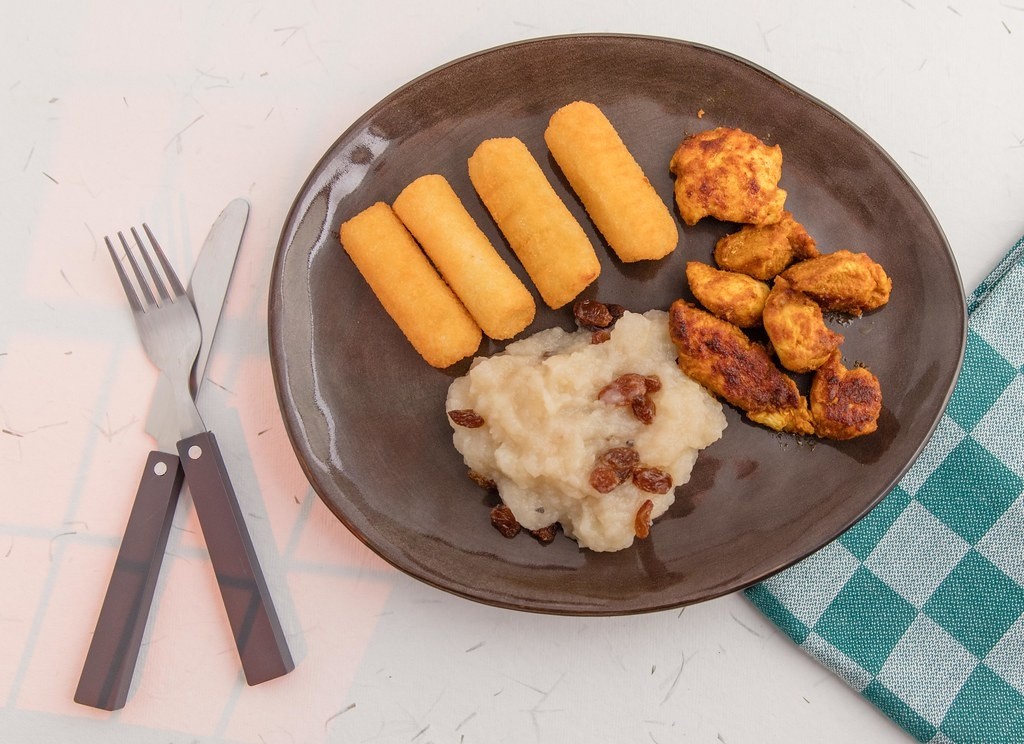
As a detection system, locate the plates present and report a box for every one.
[267,29,968,622]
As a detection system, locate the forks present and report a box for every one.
[102,225,296,690]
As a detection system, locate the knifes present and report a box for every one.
[73,194,251,710]
